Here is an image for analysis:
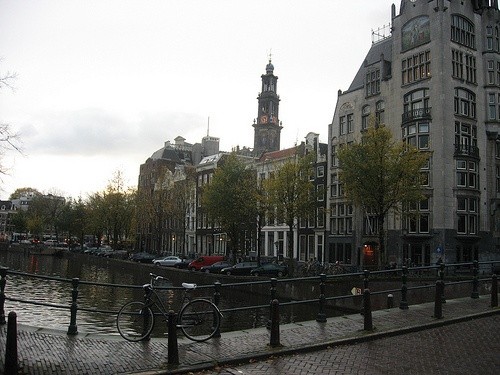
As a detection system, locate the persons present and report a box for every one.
[435,257,443,277]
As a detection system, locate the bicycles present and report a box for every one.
[114,270,224,343]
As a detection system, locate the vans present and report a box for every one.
[187,256,224,271]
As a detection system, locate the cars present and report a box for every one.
[220,262,259,275]
[250,264,287,278]
[14,237,87,251]
[88,247,157,263]
[201,261,234,273]
[153,256,185,267]
[174,258,196,269]
